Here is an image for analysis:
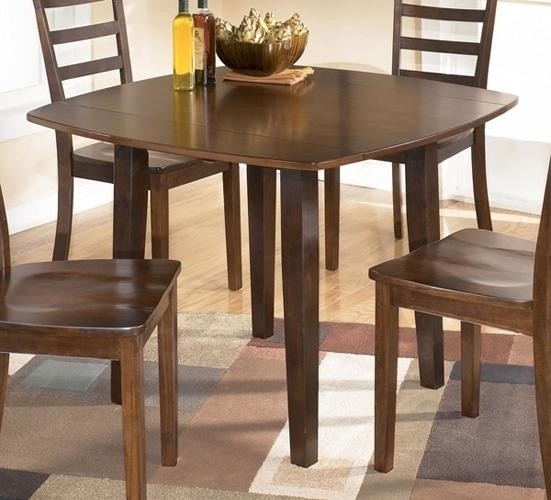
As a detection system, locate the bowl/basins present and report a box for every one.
[216,32,312,78]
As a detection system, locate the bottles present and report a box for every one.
[174,88,217,152]
[172,0,215,93]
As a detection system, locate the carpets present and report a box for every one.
[0,296,551,500]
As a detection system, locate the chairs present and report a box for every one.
[29,1,243,300]
[322,0,500,276]
[1,184,188,500]
[364,154,551,499]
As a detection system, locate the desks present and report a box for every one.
[25,60,524,474]
